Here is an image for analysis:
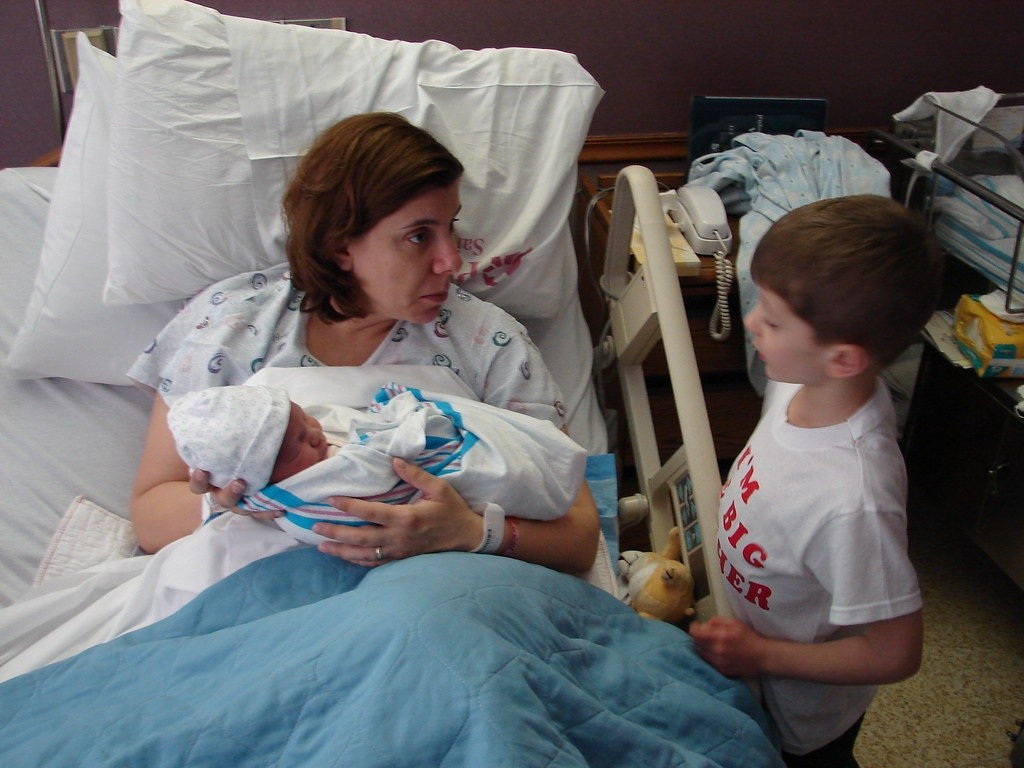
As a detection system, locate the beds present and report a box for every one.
[867,94,1024,590]
[0,163,776,768]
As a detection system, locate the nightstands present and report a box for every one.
[581,171,762,464]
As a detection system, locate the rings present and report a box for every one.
[375,547,383,560]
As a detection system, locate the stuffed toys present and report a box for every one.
[618,527,694,623]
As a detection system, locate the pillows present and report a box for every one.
[0,31,598,422]
[105,0,603,323]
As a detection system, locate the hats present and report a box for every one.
[167,385,292,492]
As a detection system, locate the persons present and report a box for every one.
[125,112,601,571]
[691,195,944,768]
[167,386,587,545]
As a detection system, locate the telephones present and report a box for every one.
[670,185,733,256]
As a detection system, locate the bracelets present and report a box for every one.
[470,503,505,553]
[209,494,229,512]
[503,519,521,556]
